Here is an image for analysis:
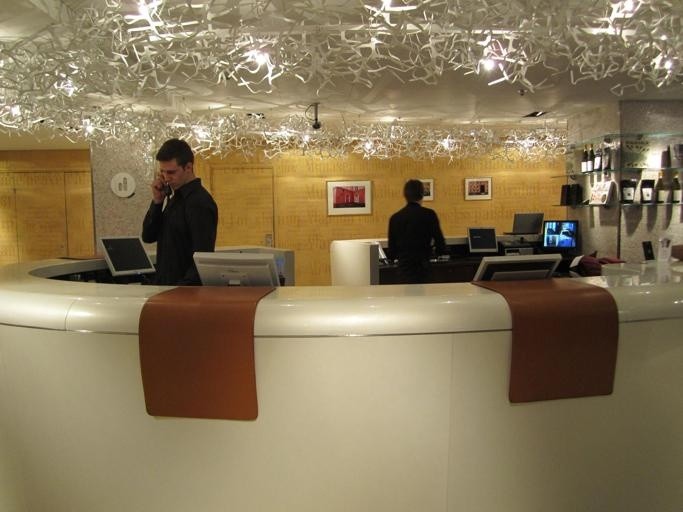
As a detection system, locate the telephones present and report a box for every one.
[162,184,172,196]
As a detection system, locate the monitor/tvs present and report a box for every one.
[468,227,498,253]
[193,252,280,286]
[98,235,155,277]
[542,220,579,256]
[473,254,562,281]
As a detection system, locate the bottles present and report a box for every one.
[670,171,681,202]
[655,172,665,204]
[580,140,611,173]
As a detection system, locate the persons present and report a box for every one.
[387,179,447,281]
[141,139,218,284]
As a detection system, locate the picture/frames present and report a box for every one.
[326,179,374,216]
[464,177,492,200]
[407,179,435,202]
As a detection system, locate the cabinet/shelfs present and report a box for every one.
[621,130,683,207]
[551,132,618,207]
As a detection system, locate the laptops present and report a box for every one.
[503,212,544,235]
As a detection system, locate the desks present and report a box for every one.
[378,253,578,284]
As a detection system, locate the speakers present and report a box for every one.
[643,241,654,260]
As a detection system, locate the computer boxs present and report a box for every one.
[500,241,533,256]
